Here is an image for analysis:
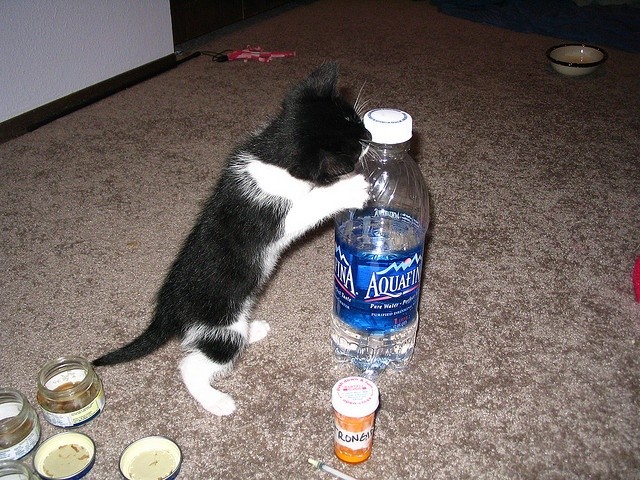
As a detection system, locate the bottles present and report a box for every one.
[329,106,431,378]
[330,375,380,463]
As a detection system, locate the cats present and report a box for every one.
[88,59,372,417]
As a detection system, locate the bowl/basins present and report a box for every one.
[547,44,607,77]
